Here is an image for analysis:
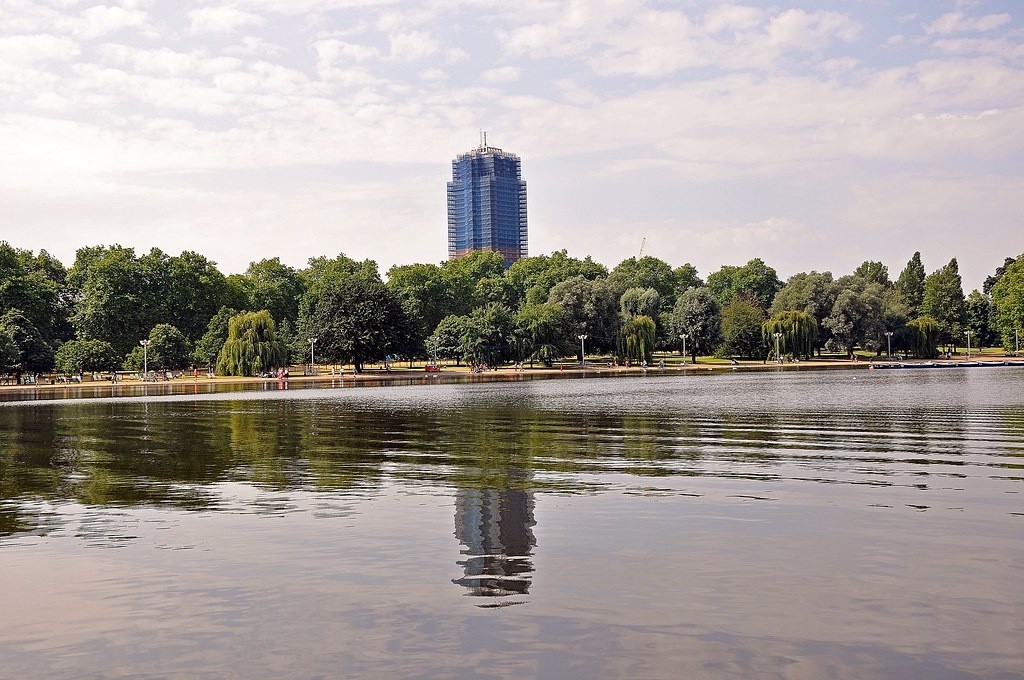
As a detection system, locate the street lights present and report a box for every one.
[883,331,893,358]
[680,334,689,365]
[1015,329,1021,352]
[140,339,151,382]
[430,336,439,368]
[964,330,973,355]
[308,337,317,374]
[577,335,588,366]
[773,333,783,360]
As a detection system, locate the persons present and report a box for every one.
[4,368,83,385]
[332,361,524,378]
[111,369,290,385]
[607,358,739,369]
[780,349,970,365]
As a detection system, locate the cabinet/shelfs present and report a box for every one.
[426,365,440,372]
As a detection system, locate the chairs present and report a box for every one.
[205,372,213,379]
[130,374,134,379]
[210,373,217,378]
[117,374,122,381]
[92,375,98,380]
[98,375,103,380]
[166,372,173,378]
[30,377,36,383]
[135,374,139,380]
